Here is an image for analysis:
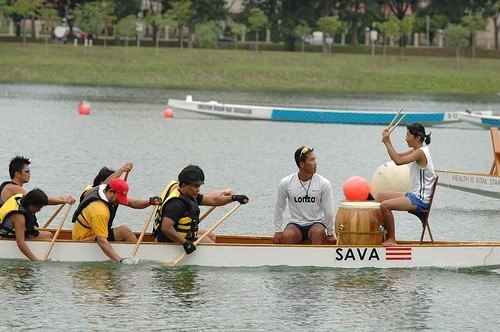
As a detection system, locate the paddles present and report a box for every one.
[124,162,133,181]
[130,197,159,258]
[43,195,71,228]
[199,191,230,222]
[42,201,75,260]
[173,199,248,265]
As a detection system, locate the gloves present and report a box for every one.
[149,195,163,206]
[183,240,196,255]
[231,194,249,205]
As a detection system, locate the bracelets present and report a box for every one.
[59,195,63,201]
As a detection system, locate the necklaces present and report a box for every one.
[298,171,313,198]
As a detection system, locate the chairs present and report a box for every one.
[408,174,439,244]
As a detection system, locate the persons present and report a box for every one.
[375,122,436,246]
[153,165,249,255]
[79,161,134,228]
[272,146,337,245]
[71,178,164,265]
[0,156,71,208]
[0,188,76,261]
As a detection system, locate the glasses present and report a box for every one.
[23,167,31,174]
[300,146,314,158]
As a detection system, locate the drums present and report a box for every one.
[335,201,387,244]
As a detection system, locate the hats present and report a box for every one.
[177,168,204,185]
[109,177,129,206]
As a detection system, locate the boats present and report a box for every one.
[167,94,493,126]
[0,226,500,269]
[453,110,500,131]
